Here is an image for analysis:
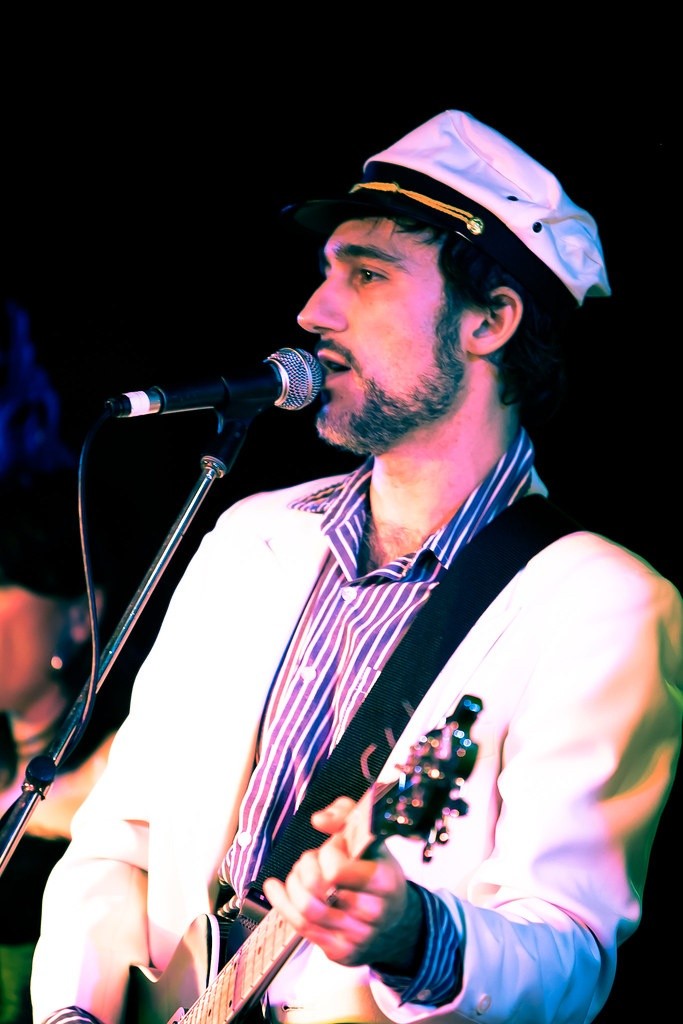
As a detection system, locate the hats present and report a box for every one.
[348,109,612,307]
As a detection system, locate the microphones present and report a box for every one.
[106,346,325,416]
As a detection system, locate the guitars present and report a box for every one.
[169,693,485,1024]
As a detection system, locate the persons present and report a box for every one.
[0,450,128,1024]
[26,103,683,1024]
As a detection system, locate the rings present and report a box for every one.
[324,888,337,906]
